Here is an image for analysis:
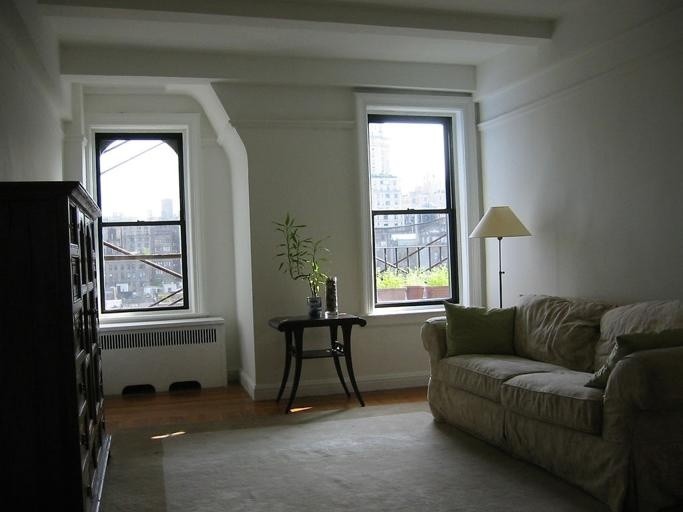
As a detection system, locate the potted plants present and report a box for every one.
[271,210,332,316]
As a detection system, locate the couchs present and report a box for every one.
[420,294,683,512]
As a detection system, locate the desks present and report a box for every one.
[268,315,368,414]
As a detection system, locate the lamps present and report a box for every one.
[468,206,533,308]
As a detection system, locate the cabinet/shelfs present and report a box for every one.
[0,180,113,512]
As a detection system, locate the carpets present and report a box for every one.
[161,412,612,512]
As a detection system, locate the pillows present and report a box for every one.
[585,334,682,388]
[442,300,517,357]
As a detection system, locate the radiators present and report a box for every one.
[100,316,227,396]
